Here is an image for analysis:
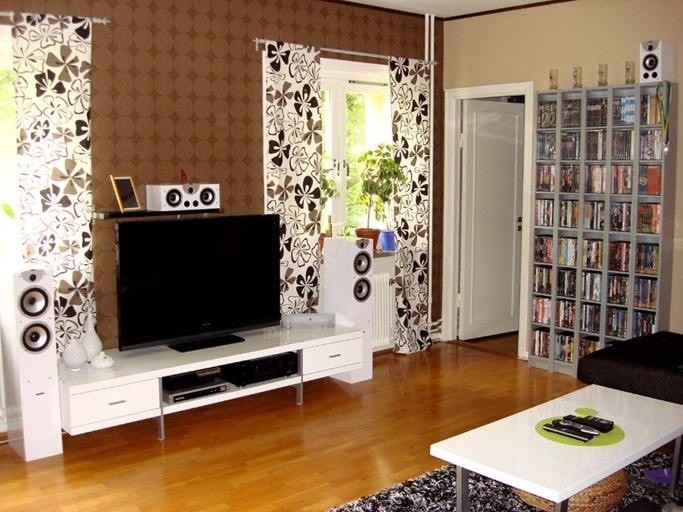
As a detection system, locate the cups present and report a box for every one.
[625,60,635,85]
[573,66,582,87]
[598,63,608,86]
[381,231,394,251]
[548,69,559,89]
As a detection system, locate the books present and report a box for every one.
[532,296,656,340]
[533,267,658,311]
[536,94,660,128]
[537,165,661,195]
[535,198,661,235]
[531,327,599,364]
[537,130,660,161]
[532,235,659,277]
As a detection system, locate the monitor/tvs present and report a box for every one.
[115,213,282,354]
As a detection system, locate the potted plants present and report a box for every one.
[353,143,408,253]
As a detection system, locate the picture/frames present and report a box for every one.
[109,173,141,215]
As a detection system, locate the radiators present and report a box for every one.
[368,273,396,353]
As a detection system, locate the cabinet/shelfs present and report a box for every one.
[529,81,678,383]
[58,316,364,439]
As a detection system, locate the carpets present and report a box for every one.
[321,450,683,512]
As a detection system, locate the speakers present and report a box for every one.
[323,237,376,386]
[145,183,222,211]
[639,40,675,83]
[0,262,65,464]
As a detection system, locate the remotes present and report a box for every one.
[586,414,614,425]
[564,413,610,433]
[541,423,591,443]
[553,418,599,436]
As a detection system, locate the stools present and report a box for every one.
[575,329,682,406]
